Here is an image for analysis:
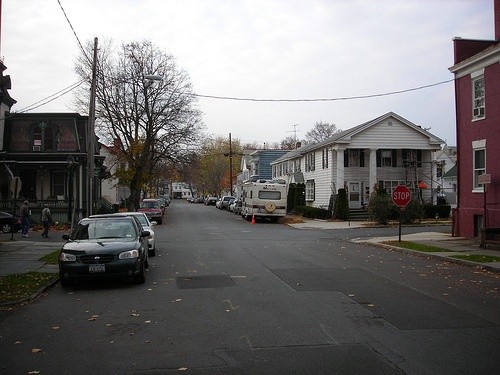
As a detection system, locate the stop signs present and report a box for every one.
[392,185,412,208]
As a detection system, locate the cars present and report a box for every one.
[216,196,243,215]
[0,210,22,233]
[139,194,172,224]
[186,197,219,206]
[59,212,158,288]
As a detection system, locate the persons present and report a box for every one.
[20,201,30,237]
[40,204,52,238]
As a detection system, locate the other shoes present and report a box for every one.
[22,233,28,237]
[40,233,50,238]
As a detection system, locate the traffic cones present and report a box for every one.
[250,214,256,224]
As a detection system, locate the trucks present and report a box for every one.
[241,178,288,223]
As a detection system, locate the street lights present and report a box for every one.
[87,75,165,219]
